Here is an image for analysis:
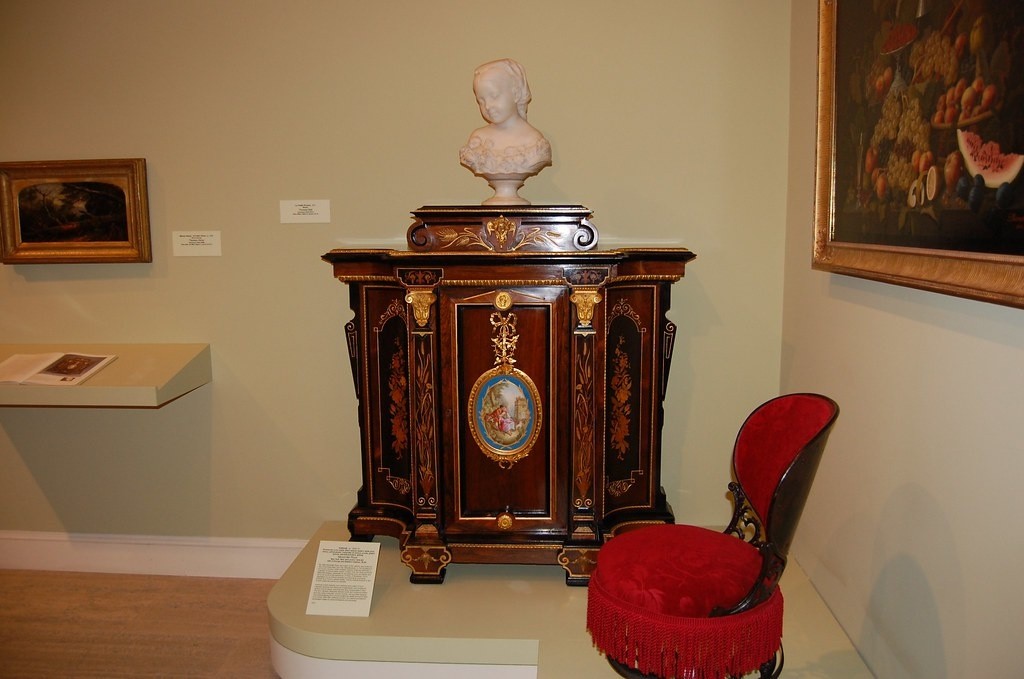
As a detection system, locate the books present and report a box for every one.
[0,352,119,387]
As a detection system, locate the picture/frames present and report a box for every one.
[812,0,1024,309]
[0,158,154,265]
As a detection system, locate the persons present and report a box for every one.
[459,58,552,177]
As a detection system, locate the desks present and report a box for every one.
[317,202,696,590]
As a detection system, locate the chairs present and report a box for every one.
[585,387,839,679]
